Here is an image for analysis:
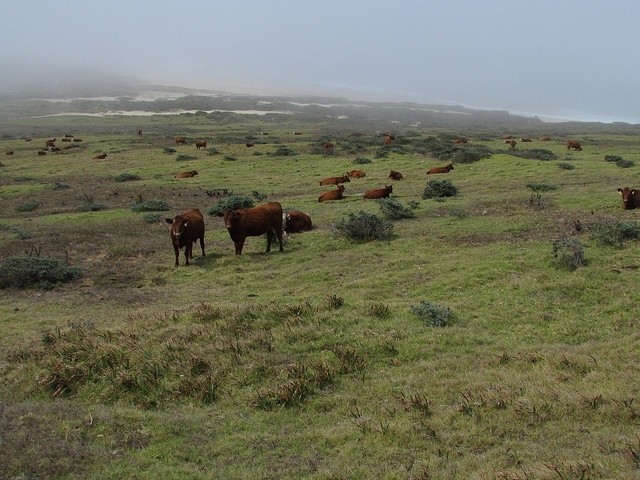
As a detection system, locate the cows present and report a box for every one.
[216,201,283,257]
[246,143,254,148]
[64,132,73,137]
[276,209,312,233]
[48,145,62,153]
[136,129,144,138]
[520,137,532,142]
[320,174,350,187]
[453,139,468,145]
[36,150,47,156]
[363,185,393,201]
[93,152,108,160]
[511,140,517,148]
[318,184,345,202]
[45,138,57,149]
[174,170,199,179]
[616,185,640,211]
[194,140,207,150]
[566,139,583,152]
[504,139,516,144]
[382,136,392,148]
[539,136,551,142]
[346,169,367,179]
[323,142,334,154]
[72,138,83,143]
[175,136,188,146]
[426,163,455,175]
[166,207,206,267]
[4,149,15,156]
[389,170,403,180]
[60,137,71,143]
